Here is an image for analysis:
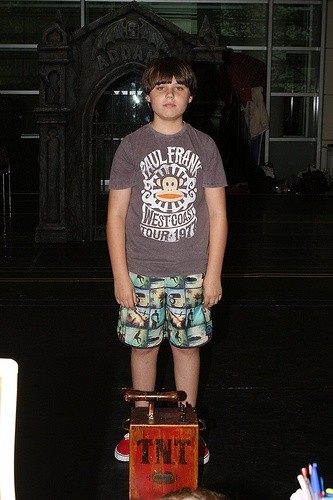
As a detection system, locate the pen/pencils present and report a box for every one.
[297,463,333,500]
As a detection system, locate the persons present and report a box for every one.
[108,56,226,464]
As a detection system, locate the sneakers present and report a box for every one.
[198,435,210,464]
[115,432,130,462]
